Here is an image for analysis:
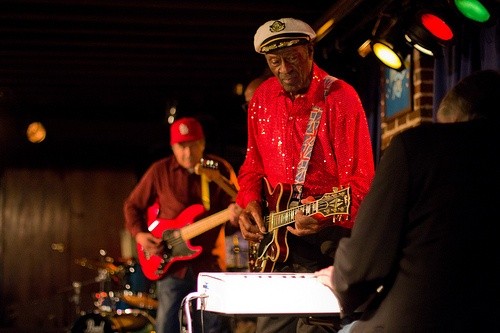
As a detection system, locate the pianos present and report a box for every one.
[183,271,341,333]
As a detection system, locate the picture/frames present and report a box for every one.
[383,52,414,123]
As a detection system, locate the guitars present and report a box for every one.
[137,204,243,280]
[248,176,353,273]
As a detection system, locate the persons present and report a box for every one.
[238,18,375,333]
[332,68,500,333]
[123,117,239,333]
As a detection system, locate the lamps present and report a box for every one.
[369,0,500,73]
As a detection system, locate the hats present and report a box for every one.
[254,18,317,54]
[170,118,204,145]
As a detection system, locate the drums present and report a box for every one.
[72,256,148,333]
[117,256,160,308]
